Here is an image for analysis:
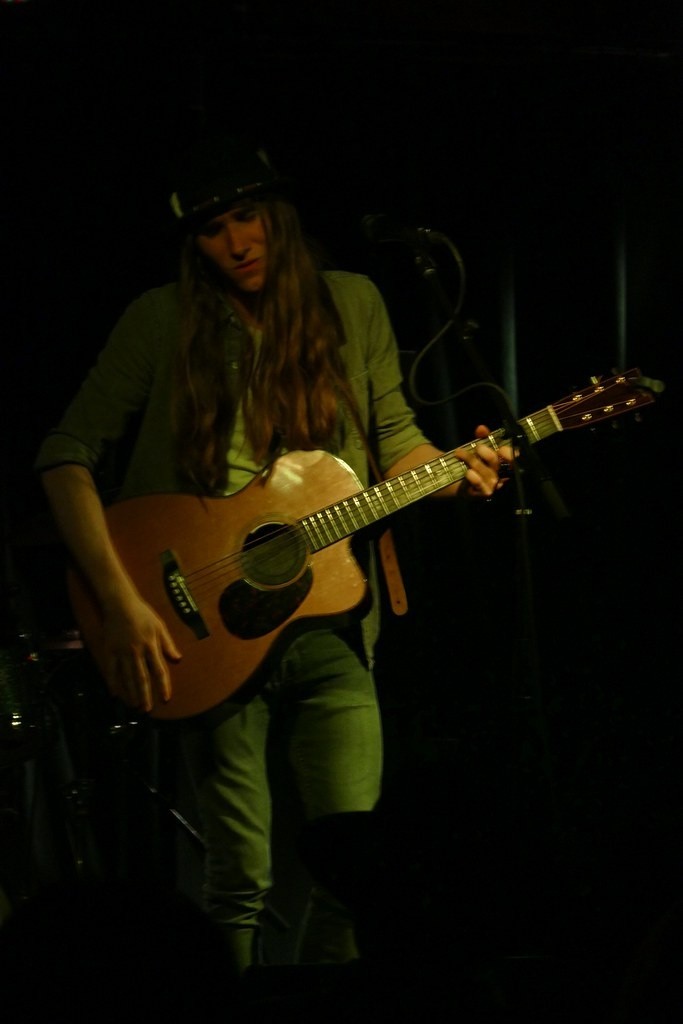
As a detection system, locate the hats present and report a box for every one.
[169,143,276,225]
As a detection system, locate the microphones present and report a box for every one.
[362,213,447,245]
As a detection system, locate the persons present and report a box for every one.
[33,137,522,1006]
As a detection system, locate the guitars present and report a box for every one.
[67,368,669,733]
